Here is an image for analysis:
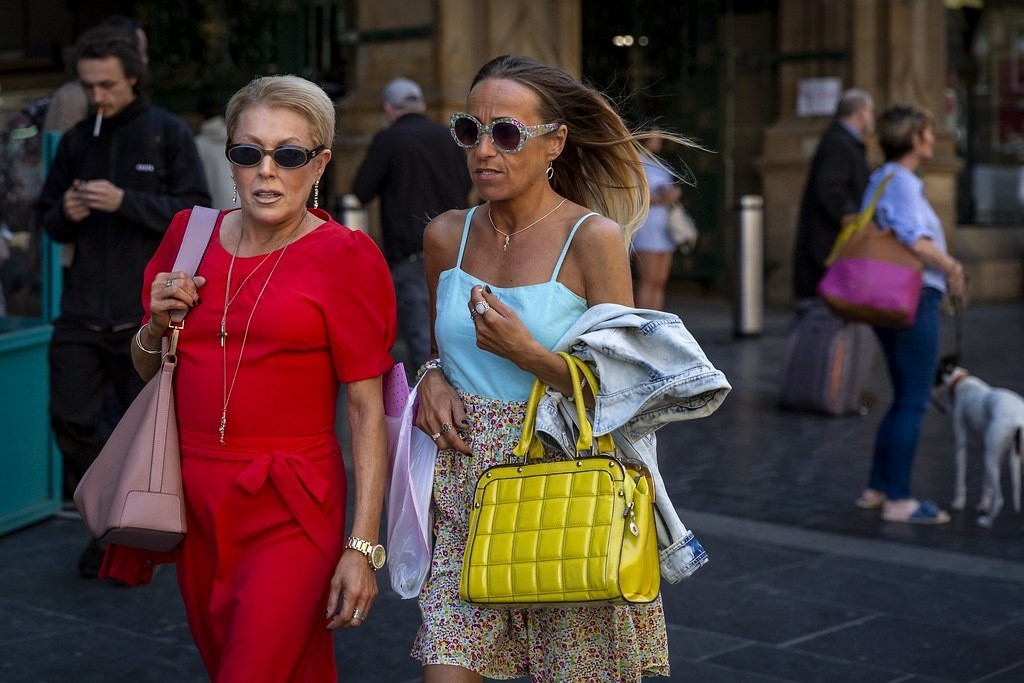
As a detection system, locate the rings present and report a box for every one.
[352,608,360,619]
[439,421,452,433]
[469,309,478,319]
[475,300,490,315]
[165,277,174,286]
[432,431,441,441]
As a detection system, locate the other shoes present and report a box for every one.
[79,538,104,577]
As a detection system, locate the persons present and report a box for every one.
[41,20,149,281]
[860,105,966,526]
[795,88,874,302]
[354,77,473,390]
[628,121,680,310]
[421,53,649,683]
[130,76,398,681]
[42,28,214,588]
[183,83,242,211]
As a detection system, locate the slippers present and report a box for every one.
[856,489,886,509]
[882,497,952,524]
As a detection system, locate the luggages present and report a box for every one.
[782,304,878,416]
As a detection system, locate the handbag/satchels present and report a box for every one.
[382,362,444,598]
[668,199,697,255]
[73,206,219,550]
[819,173,924,326]
[20,95,53,129]
[459,352,661,608]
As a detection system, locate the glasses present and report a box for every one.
[448,114,561,155]
[224,138,325,170]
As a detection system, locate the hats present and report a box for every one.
[380,78,422,105]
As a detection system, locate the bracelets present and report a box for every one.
[135,322,161,355]
[415,357,441,380]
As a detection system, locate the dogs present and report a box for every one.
[939,363,1024,527]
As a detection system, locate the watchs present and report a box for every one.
[342,534,387,571]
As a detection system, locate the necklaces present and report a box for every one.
[487,196,569,251]
[217,199,307,447]
